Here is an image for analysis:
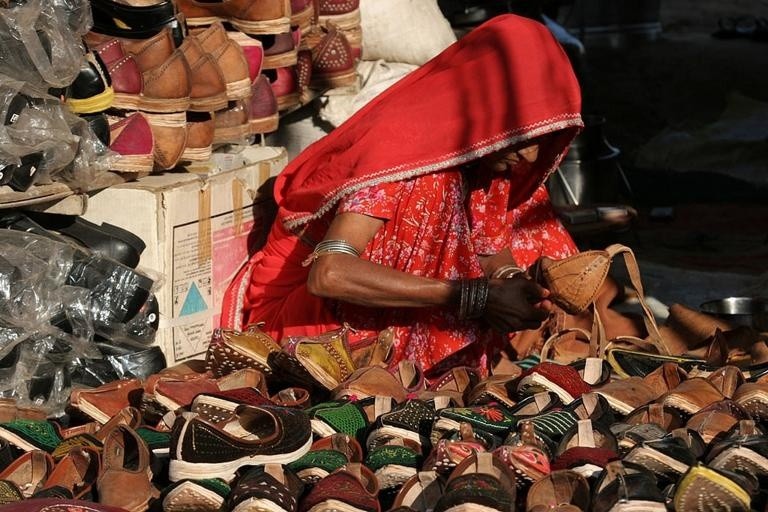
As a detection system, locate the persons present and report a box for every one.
[222,12,583,388]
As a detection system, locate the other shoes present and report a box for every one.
[0,321,768,512]
[0,0,225,193]
[0,205,166,398]
[199,0,362,144]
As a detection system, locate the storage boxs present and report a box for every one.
[88,144,291,370]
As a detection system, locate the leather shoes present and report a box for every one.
[0,206,166,405]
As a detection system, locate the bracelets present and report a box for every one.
[460,276,488,322]
[489,263,524,281]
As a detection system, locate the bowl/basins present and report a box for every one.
[699,296,768,333]
